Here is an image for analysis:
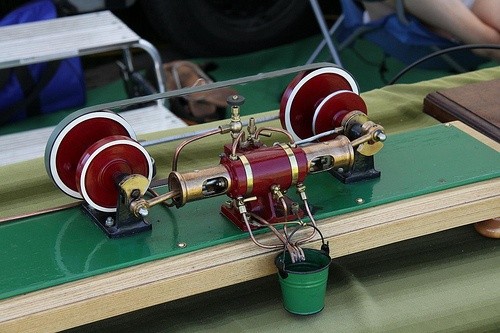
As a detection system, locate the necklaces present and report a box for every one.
[340,0,500,60]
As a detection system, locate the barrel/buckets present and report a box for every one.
[274,224,332,316]
